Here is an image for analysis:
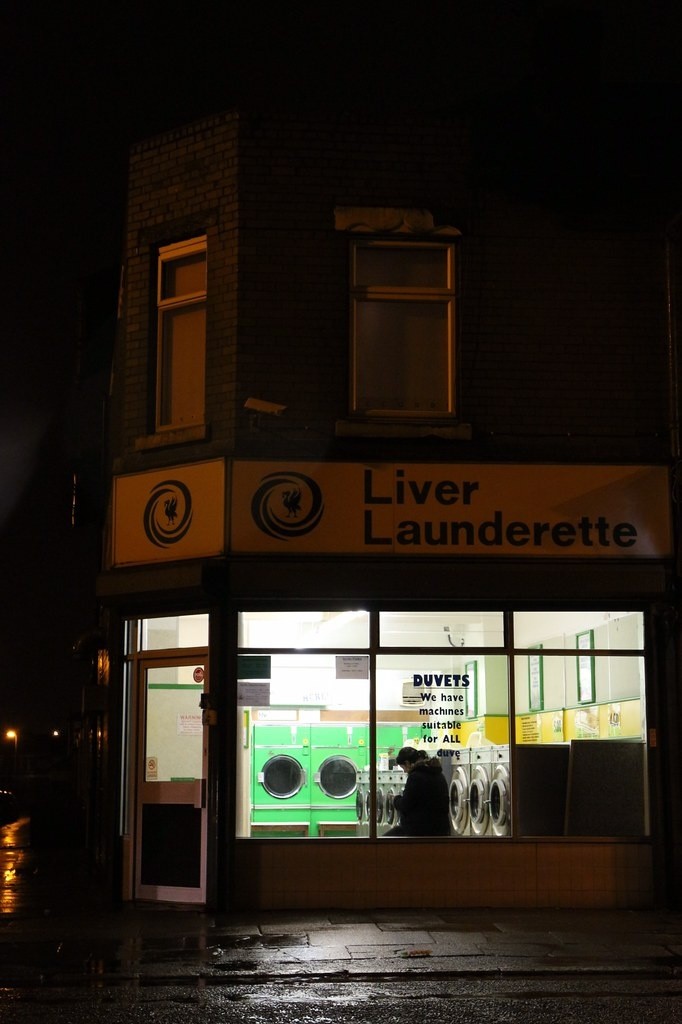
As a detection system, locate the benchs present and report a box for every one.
[248,821,309,839]
[315,820,359,838]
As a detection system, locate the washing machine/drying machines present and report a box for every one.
[372,720,430,771]
[309,720,369,823]
[249,723,311,823]
[356,740,570,838]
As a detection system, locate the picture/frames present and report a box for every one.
[574,629,596,705]
[463,660,478,719]
[526,644,546,712]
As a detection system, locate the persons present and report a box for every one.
[381,747,451,837]
